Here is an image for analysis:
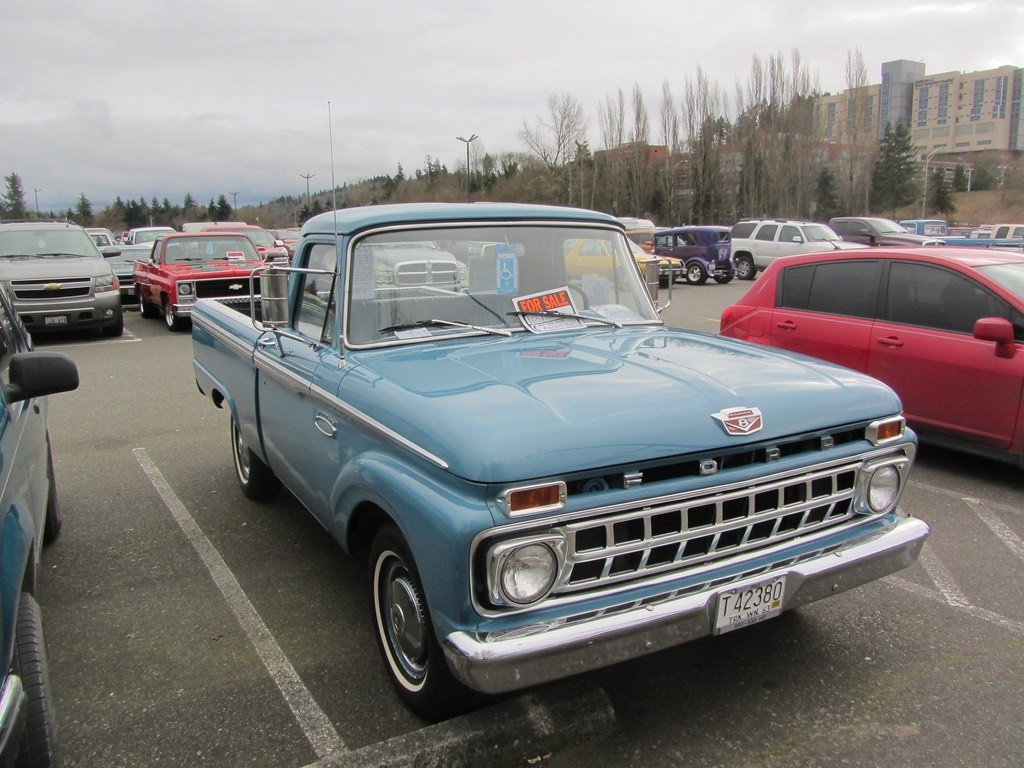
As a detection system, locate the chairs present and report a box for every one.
[939,275,977,335]
[215,242,238,259]
[166,244,185,263]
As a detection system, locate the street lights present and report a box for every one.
[298,173,315,219]
[455,133,478,202]
[957,157,971,192]
[228,190,241,222]
[33,187,41,219]
[921,146,953,219]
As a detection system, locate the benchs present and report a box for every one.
[358,291,517,339]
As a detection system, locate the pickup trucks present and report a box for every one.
[191,201,930,724]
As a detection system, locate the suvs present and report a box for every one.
[828,217,946,248]
[729,217,869,280]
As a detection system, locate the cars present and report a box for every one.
[318,240,470,295]
[719,247,1024,470]
[561,217,739,289]
[899,219,1024,249]
[0,222,300,339]
[0,277,82,767]
